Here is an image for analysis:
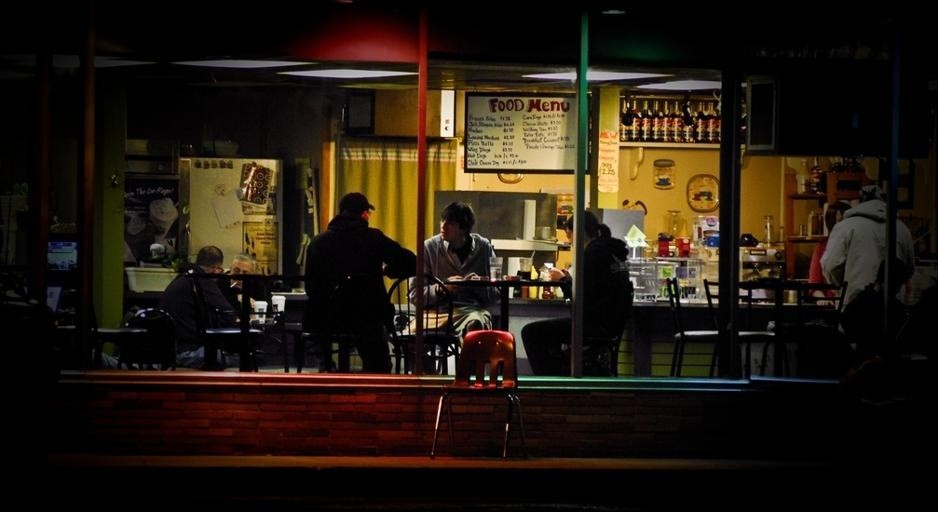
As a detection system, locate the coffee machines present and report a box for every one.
[739,246,787,300]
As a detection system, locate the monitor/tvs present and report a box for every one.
[46,241,81,272]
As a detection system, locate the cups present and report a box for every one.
[489,256,504,280]
[542,227,551,240]
[656,237,669,257]
[519,258,532,273]
[271,295,287,324]
[253,301,269,324]
[521,286,543,299]
[675,238,690,257]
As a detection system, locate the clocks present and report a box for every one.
[686,174,722,212]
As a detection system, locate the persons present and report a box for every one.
[305,193,416,374]
[217,253,257,331]
[521,211,634,377]
[819,185,915,333]
[403,201,500,376]
[807,201,853,306]
[156,246,240,372]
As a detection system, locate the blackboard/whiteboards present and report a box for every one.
[464,91,592,174]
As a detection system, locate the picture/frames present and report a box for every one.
[497,173,524,184]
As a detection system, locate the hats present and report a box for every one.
[339,192,375,215]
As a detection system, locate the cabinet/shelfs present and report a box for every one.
[782,165,867,277]
[613,94,724,152]
[373,90,464,139]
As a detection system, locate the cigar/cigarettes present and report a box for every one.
[230,280,239,288]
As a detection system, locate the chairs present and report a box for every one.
[427,328,528,459]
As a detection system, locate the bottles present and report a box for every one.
[620,97,721,143]
[663,209,683,237]
[796,161,810,194]
[807,207,823,236]
[762,215,775,249]
[799,224,806,236]
[810,155,827,195]
